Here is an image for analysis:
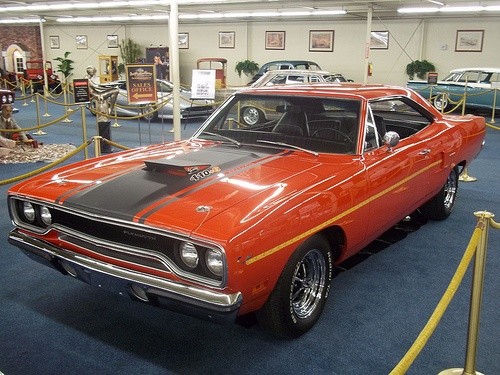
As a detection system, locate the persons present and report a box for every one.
[136,59,142,63]
[153,50,169,79]
[84,67,119,114]
[0,105,38,148]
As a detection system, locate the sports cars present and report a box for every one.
[89,78,216,124]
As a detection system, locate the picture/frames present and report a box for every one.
[455,30,483,51]
[309,30,334,51]
[370,31,389,48]
[265,31,285,50]
[49,36,59,48]
[107,35,118,48]
[76,35,87,48]
[219,31,235,47]
[178,33,189,48]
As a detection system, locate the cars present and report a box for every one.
[227,67,397,130]
[406,68,500,115]
[0,89,19,114]
[6,84,486,339]
[244,59,336,84]
[19,60,62,95]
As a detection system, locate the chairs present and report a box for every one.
[276,114,386,147]
